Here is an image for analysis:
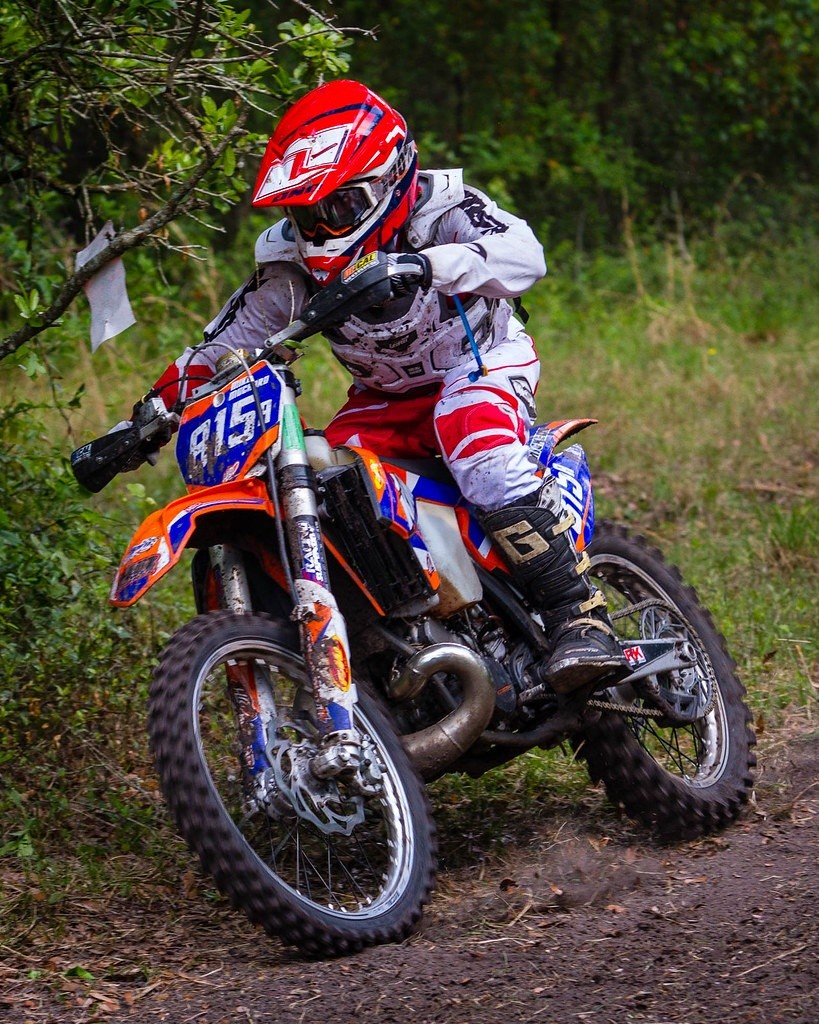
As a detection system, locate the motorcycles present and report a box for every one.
[70,251,758,962]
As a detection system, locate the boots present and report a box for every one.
[478,473,632,693]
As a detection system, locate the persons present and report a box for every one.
[106,77,635,691]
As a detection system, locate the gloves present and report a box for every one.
[370,251,431,308]
[106,420,159,472]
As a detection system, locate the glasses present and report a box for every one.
[288,181,380,238]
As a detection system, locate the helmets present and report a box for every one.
[252,80,418,287]
